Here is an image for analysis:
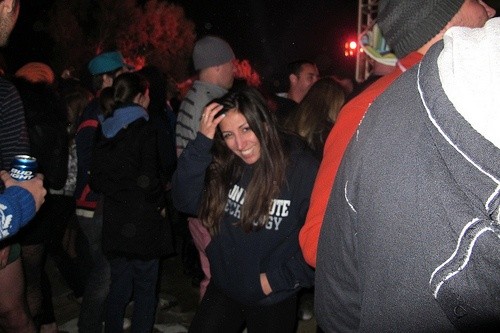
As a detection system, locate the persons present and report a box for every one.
[172,86,321,333]
[298,0,500,333]
[0,0,175,333]
[167,18,398,320]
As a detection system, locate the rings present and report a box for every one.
[202,114,205,117]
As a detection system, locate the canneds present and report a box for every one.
[10,155,36,182]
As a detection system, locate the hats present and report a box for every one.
[88,51,135,75]
[359,23,397,67]
[193,35,236,70]
[376,0,466,59]
